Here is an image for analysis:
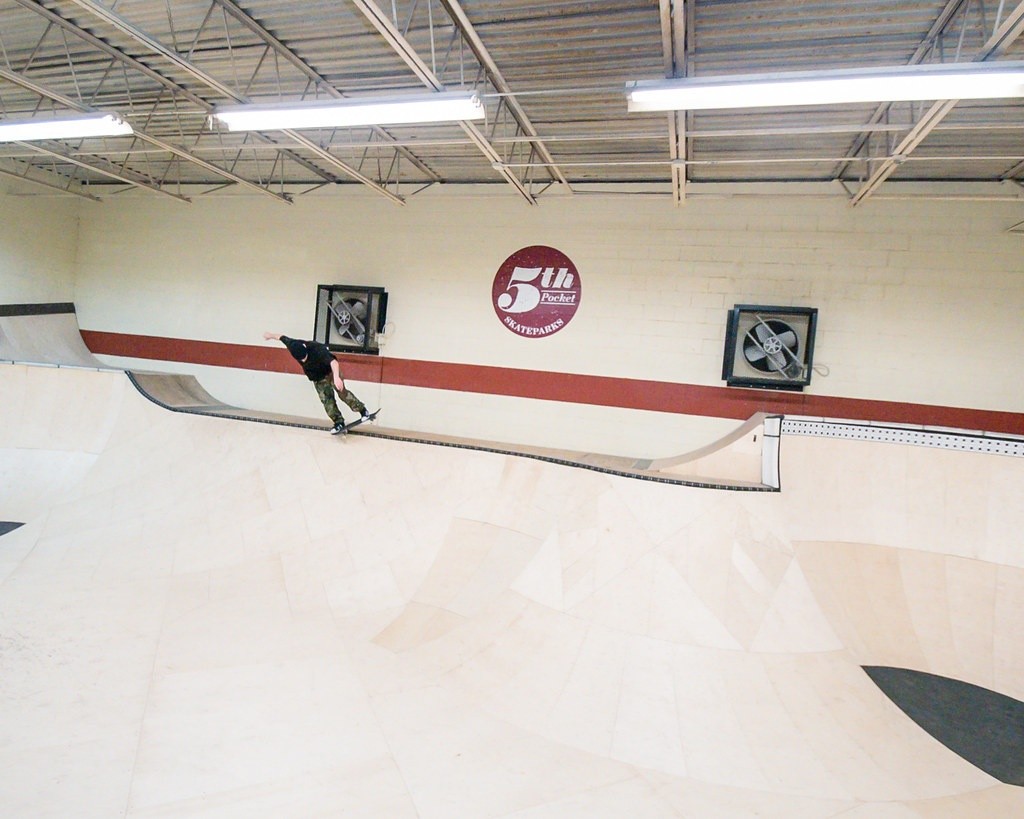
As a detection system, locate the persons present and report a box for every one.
[264,332,370,434]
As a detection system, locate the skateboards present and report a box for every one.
[330,408,381,436]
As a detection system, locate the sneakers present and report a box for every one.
[330,422,345,435]
[359,407,370,422]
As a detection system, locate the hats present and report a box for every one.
[290,342,307,360]
